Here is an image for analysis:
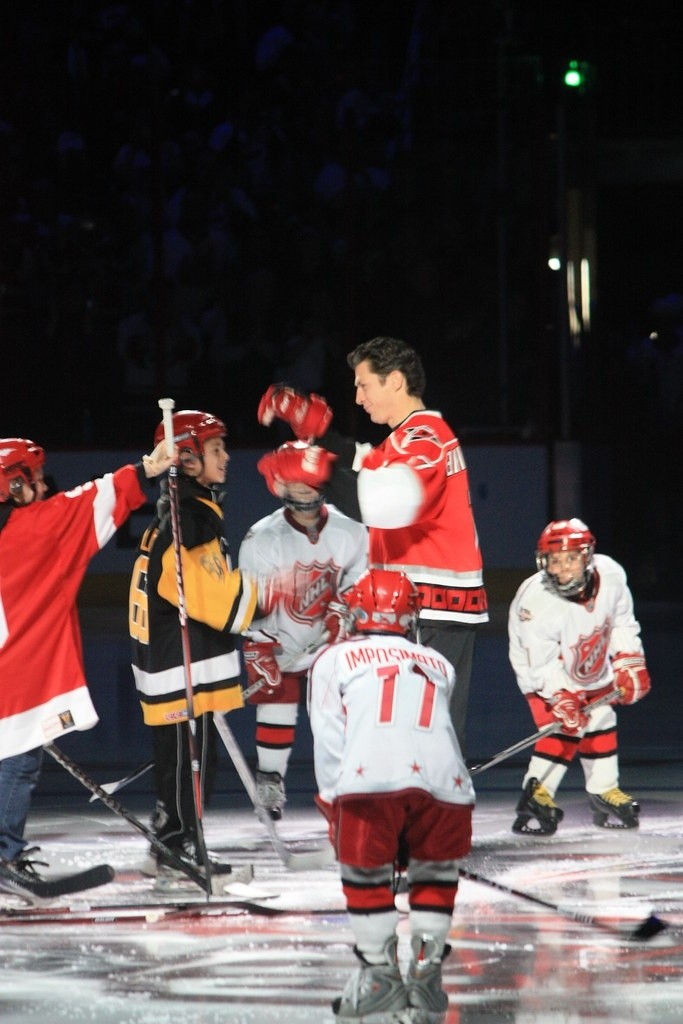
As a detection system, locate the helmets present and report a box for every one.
[535,517,596,600]
[0,438,45,502]
[347,567,421,635]
[153,410,228,467]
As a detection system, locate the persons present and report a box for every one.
[506,519,650,835]
[258,338,489,749]
[308,567,477,1024]
[0,437,180,915]
[237,439,372,828]
[127,409,289,889]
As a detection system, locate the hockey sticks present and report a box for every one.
[458,868,667,943]
[89,632,328,803]
[0,861,117,900]
[156,395,284,917]
[45,743,232,897]
[209,710,336,873]
[468,686,626,776]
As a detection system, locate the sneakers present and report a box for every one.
[138,832,220,877]
[0,856,64,914]
[404,932,449,1024]
[330,937,408,1024]
[511,778,564,836]
[153,855,237,898]
[252,764,286,824]
[587,785,641,830]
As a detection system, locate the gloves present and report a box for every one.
[271,438,339,486]
[239,642,284,705]
[544,690,590,737]
[319,595,350,645]
[607,653,651,706]
[256,382,334,441]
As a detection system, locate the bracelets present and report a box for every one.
[143,456,157,475]
